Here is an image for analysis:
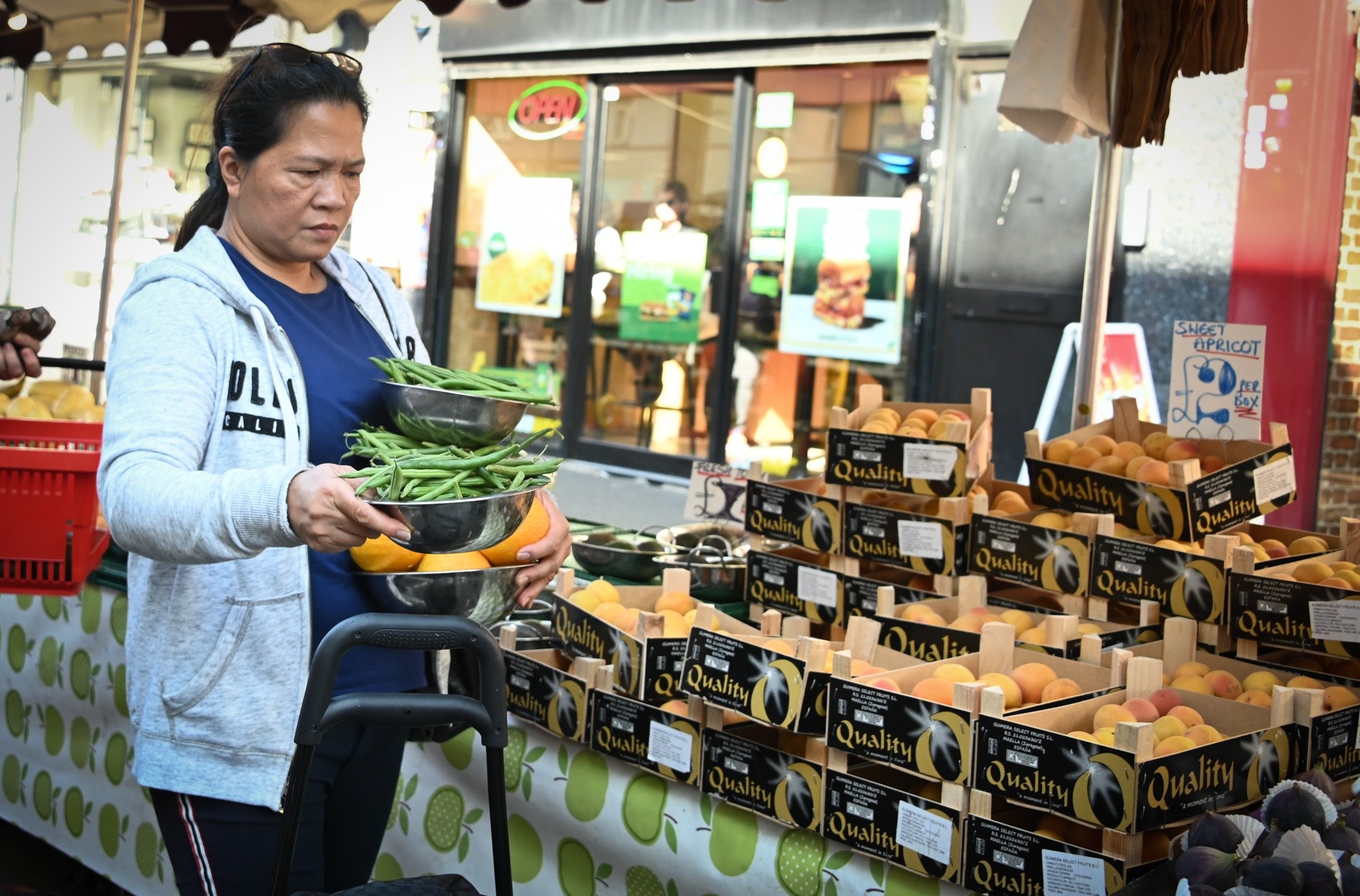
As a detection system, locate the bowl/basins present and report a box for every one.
[570,540,677,582]
[369,377,539,449]
[348,561,538,632]
[652,554,747,603]
[358,474,551,555]
[656,523,749,555]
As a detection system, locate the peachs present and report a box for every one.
[568,579,1360,862]
[814,410,1360,593]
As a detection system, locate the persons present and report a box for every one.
[0,333,41,381]
[96,42,572,896]
[539,181,785,448]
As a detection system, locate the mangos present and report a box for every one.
[0,377,105,427]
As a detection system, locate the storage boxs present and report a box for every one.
[0,419,112,596]
[497,404,1360,896]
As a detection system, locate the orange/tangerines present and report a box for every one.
[348,495,552,574]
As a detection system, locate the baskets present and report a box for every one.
[0,356,108,597]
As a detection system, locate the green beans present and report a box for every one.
[391,409,514,445]
[369,356,559,405]
[337,421,565,501]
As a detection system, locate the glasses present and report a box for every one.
[215,42,362,117]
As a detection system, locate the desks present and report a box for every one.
[0,521,977,896]
